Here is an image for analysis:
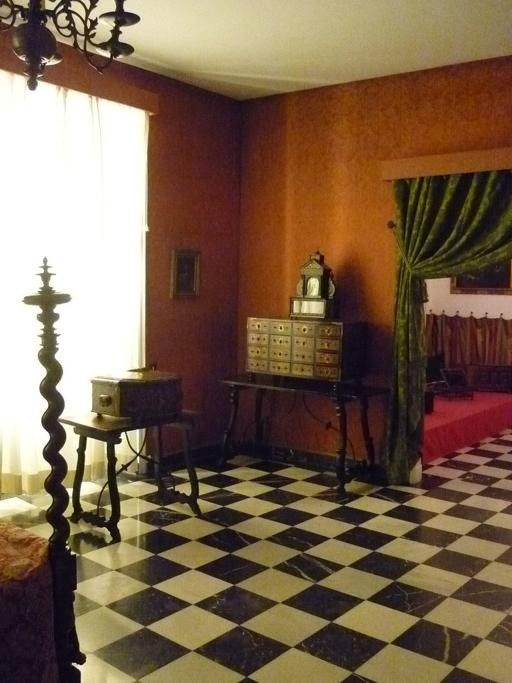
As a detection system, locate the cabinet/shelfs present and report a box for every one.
[246,317,369,392]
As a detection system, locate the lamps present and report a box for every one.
[1,0,140,91]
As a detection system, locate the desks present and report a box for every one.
[57,409,204,541]
[215,372,391,498]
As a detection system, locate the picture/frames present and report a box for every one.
[169,249,202,300]
